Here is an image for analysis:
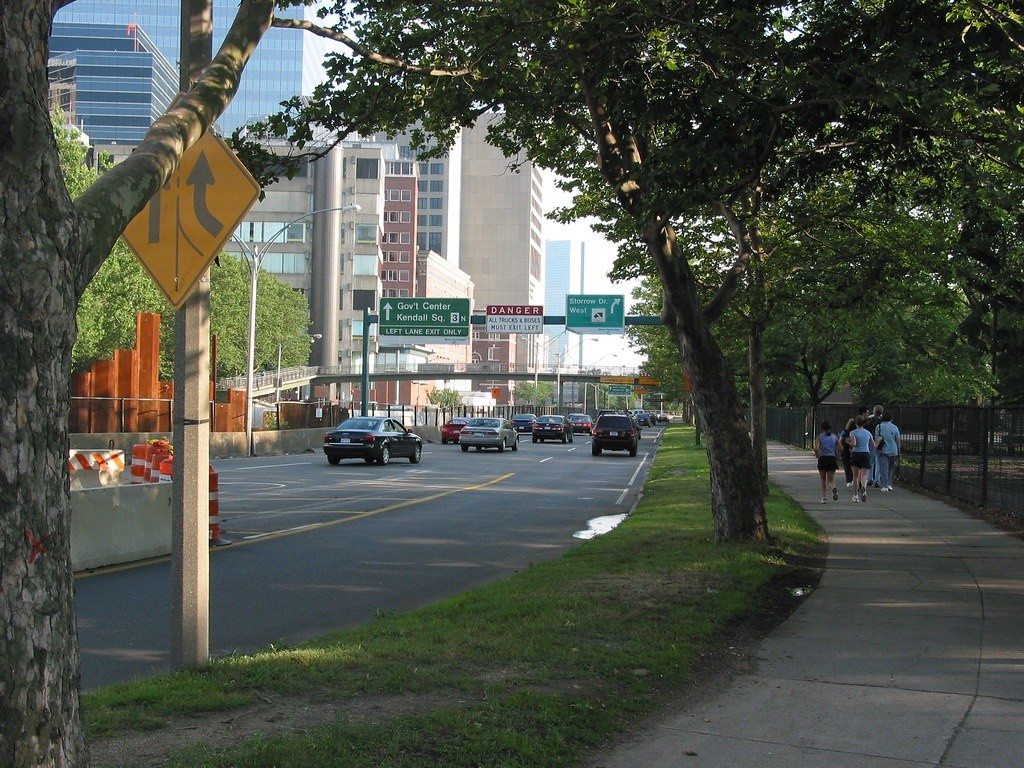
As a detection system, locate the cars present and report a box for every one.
[566,413,582,421]
[441,417,471,444]
[532,415,574,444]
[567,414,593,436]
[459,417,520,453]
[511,414,537,432]
[322,417,423,466]
[596,409,669,428]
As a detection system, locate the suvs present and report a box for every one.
[591,413,639,457]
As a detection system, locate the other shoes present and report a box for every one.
[860,487,868,502]
[874,483,879,488]
[852,495,859,502]
[867,480,873,486]
[881,487,888,492]
[847,481,853,487]
[820,498,827,504]
[888,485,893,491]
[831,488,839,501]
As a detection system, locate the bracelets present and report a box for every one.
[847,441,849,445]
[816,453,820,455]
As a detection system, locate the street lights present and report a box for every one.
[540,338,598,415]
[228,203,362,458]
[565,353,618,415]
[277,333,322,401]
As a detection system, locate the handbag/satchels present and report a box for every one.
[874,424,884,450]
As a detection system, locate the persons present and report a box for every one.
[623,408,633,419]
[875,412,901,492]
[845,415,876,503]
[839,418,857,487]
[814,421,841,503]
[857,405,870,421]
[867,405,884,488]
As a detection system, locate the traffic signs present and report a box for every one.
[565,293,625,331]
[608,384,631,397]
[377,296,470,346]
